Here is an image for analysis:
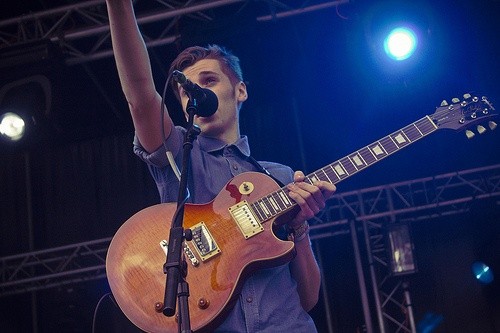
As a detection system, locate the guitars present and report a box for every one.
[106,92,500,333]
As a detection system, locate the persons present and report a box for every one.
[106,0,336,333]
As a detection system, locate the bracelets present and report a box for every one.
[288,220,310,242]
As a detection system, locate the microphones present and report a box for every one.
[173,70,218,118]
